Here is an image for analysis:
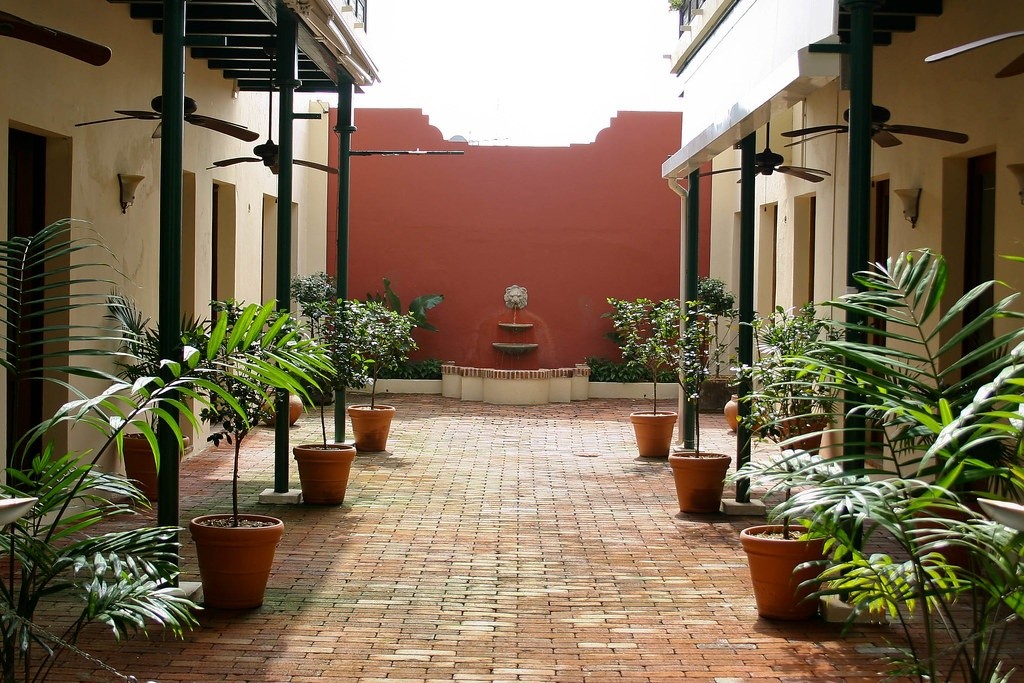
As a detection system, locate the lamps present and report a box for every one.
[893,187,923,229]
[117,174,146,214]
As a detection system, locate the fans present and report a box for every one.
[684,122,831,183]
[780,106,968,149]
[75,95,259,143]
[213,44,339,174]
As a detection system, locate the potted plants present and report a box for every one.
[287,316,357,505]
[889,342,1008,577]
[724,308,739,433]
[641,302,742,516]
[727,305,876,624]
[175,299,297,611]
[332,298,419,453]
[258,318,303,427]
[599,296,680,458]
[102,287,189,499]
[697,276,738,412]
[754,306,845,458]
